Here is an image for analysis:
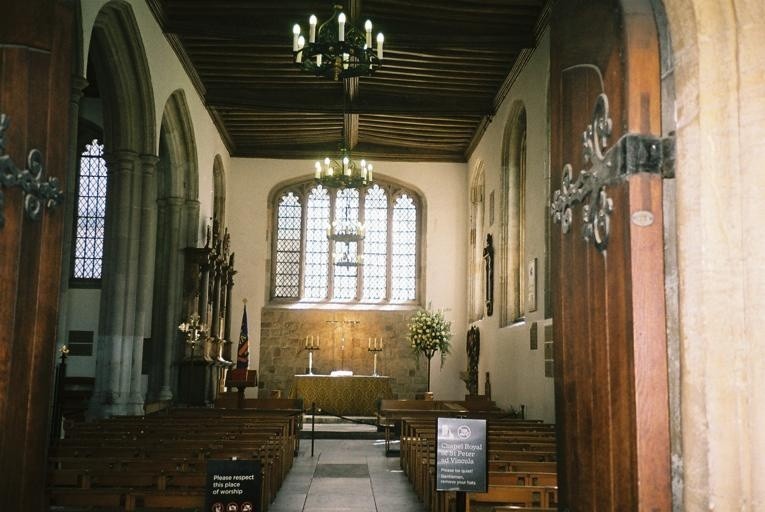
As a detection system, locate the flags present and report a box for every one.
[236,305,248,368]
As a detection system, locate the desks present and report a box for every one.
[215,399,303,451]
[380,400,468,456]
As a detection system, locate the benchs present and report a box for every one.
[44,408,302,512]
[400,417,558,512]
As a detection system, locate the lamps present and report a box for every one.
[314,128,374,270]
[292,0,384,82]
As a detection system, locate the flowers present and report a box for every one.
[401,298,455,372]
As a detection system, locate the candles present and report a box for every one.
[305,335,319,347]
[369,337,382,348]
